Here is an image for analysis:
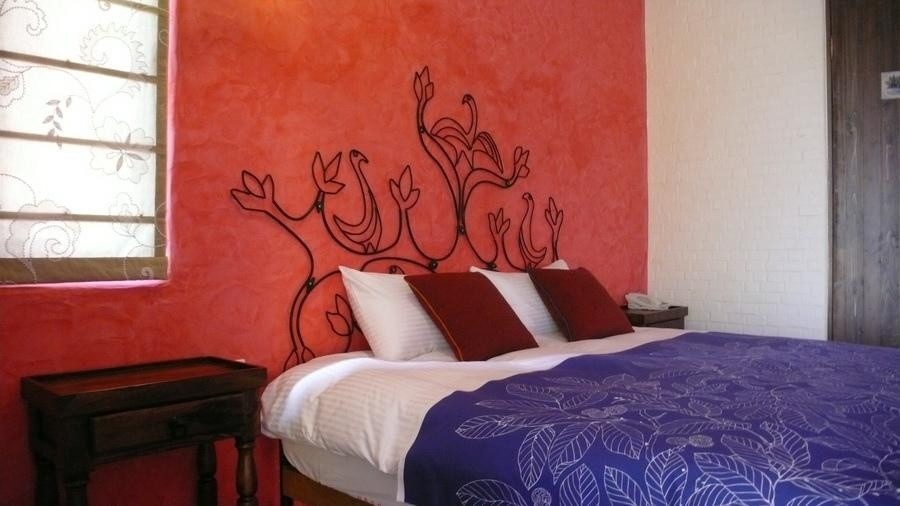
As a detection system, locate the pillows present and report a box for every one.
[336,260,634,365]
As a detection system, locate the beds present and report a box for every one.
[262,322,900,506]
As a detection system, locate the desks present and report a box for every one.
[19,352,268,504]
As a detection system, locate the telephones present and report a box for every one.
[625,292,668,311]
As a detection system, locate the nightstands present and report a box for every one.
[618,301,689,332]
